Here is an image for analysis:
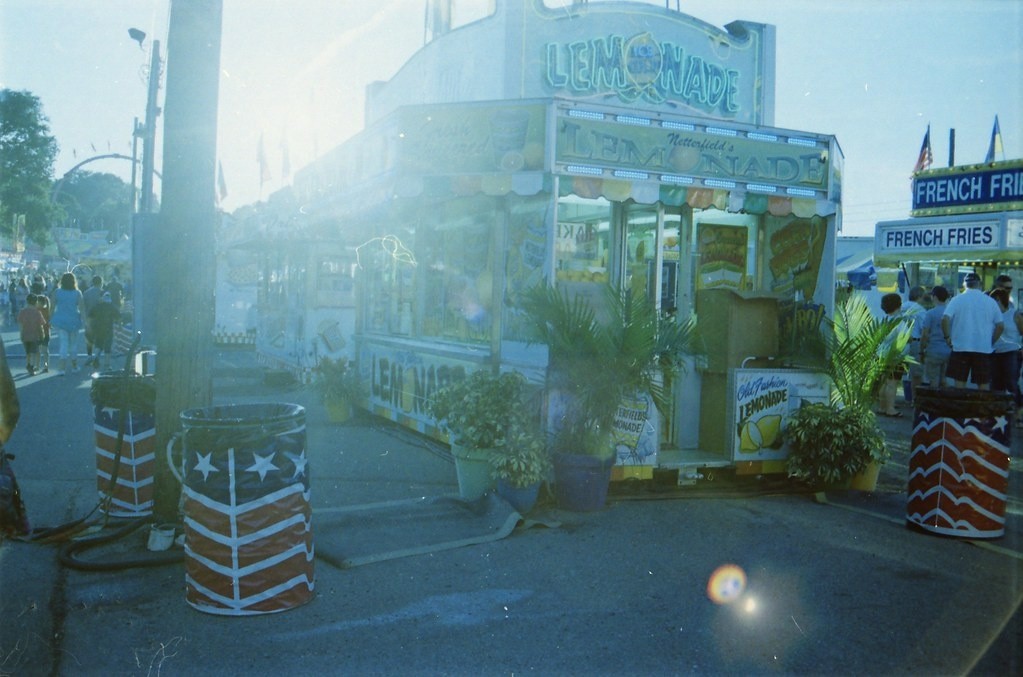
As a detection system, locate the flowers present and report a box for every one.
[301,355,367,395]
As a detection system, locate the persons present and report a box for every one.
[877,272,1023,418]
[34,294,52,372]
[0,263,123,375]
[18,294,47,376]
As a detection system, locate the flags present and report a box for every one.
[985,116,1003,162]
[910,131,933,180]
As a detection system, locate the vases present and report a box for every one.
[324,388,351,422]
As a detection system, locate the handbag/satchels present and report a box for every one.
[0,449,31,540]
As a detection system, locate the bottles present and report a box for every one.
[216,332,255,344]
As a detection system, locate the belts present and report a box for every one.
[912,337,921,341]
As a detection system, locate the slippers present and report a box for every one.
[887,412,904,418]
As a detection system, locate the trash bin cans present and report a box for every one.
[905,385,1017,538]
[90,368,156,516]
[180,402,316,615]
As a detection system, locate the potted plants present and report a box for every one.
[433,374,494,500]
[487,373,555,512]
[494,295,699,515]
[783,296,913,493]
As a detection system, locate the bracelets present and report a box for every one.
[919,353,922,354]
[944,336,949,339]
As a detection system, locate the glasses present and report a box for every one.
[999,284,1013,291]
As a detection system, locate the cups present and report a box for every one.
[490,109,529,168]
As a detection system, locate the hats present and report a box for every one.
[964,273,980,284]
[928,286,947,295]
[101,291,112,303]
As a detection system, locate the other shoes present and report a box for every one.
[898,401,914,408]
[72,366,80,373]
[26,363,35,377]
[44,364,48,372]
[58,370,65,375]
